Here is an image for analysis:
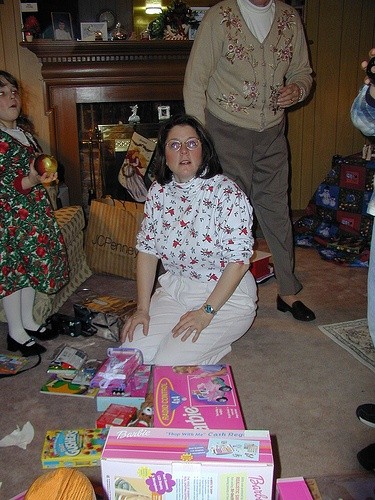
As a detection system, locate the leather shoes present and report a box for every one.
[276,293,316,321]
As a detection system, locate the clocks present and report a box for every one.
[96,9,118,31]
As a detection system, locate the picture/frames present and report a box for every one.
[51,11,74,40]
[81,22,107,41]
[188,7,210,40]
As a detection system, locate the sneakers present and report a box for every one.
[357,443,375,478]
[356,404,375,429]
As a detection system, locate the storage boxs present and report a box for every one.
[91,348,314,500]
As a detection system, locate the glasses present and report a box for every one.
[164,137,200,152]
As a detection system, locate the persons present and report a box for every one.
[184,0,317,321]
[109,115,260,365]
[0,70,68,358]
[350,47,375,428]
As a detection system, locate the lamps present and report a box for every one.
[145,0,163,14]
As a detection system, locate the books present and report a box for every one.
[39,373,99,398]
[43,427,107,470]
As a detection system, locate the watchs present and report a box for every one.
[203,303,216,316]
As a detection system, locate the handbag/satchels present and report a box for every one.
[73,288,137,342]
[85,195,145,281]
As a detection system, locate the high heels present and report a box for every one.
[7,326,59,356]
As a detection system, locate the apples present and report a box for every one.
[35,153,58,178]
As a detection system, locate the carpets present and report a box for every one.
[318,317,375,373]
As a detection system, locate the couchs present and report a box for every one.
[0,187,93,323]
